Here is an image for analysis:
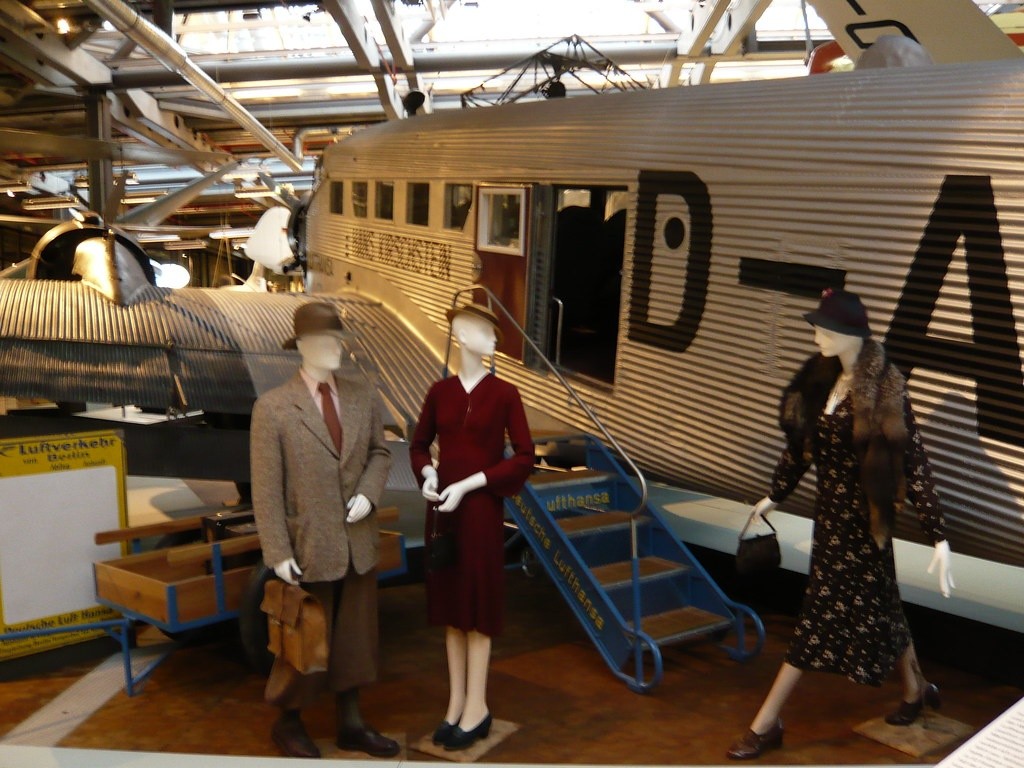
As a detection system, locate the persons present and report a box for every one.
[409,305,537,750]
[729,290,956,758]
[250,299,402,755]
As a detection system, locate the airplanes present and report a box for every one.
[1,1,1024,697]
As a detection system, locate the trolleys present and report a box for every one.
[1,520,405,697]
